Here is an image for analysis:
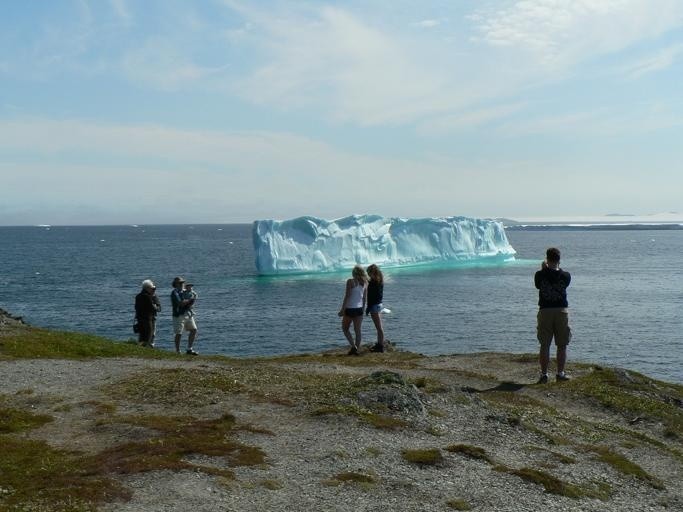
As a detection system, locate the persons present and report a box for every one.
[150,286,161,348]
[368,265,385,351]
[172,276,200,355]
[183,283,198,318]
[135,279,154,345]
[535,247,571,384]
[338,265,368,355]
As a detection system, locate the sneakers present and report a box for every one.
[187,350,199,355]
[348,344,384,356]
[556,373,571,381]
[536,376,547,384]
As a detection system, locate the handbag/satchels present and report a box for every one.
[132,317,139,333]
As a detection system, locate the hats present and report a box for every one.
[184,282,193,287]
[173,276,186,284]
